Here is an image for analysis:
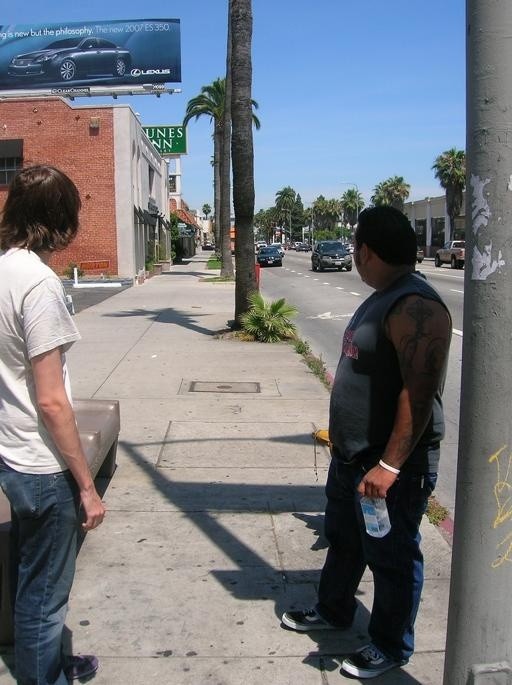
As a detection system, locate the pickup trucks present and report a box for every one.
[434,240,465,269]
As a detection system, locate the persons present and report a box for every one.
[0,162,108,684]
[280,205,455,682]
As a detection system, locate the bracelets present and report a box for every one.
[377,457,403,476]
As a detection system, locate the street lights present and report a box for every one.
[254,224,266,242]
[339,181,361,223]
[281,208,291,240]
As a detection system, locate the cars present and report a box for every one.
[7,35,134,82]
[254,239,312,268]
[310,241,353,272]
[343,242,355,254]
[415,245,426,263]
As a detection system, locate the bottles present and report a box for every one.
[358,496,391,540]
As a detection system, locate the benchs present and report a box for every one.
[1,397,125,645]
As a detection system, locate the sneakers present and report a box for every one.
[282,607,341,632]
[61,654,99,681]
[341,642,408,678]
